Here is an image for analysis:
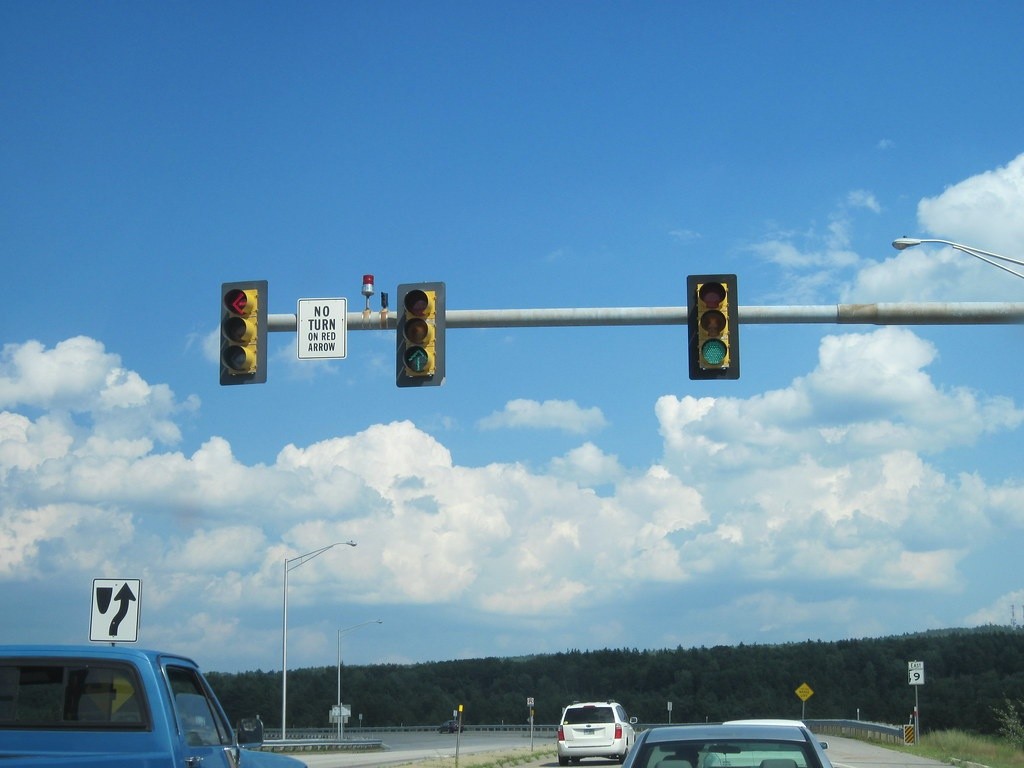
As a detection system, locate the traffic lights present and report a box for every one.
[395,281,446,389]
[219,280,269,386]
[685,273,742,381]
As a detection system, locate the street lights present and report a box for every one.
[279,540,358,742]
[335,618,383,742]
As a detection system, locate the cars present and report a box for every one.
[555,699,636,764]
[621,722,835,768]
[703,717,812,768]
[438,720,464,734]
[1,643,308,768]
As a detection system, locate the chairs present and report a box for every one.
[759,758,799,768]
[654,759,692,768]
[663,755,695,767]
